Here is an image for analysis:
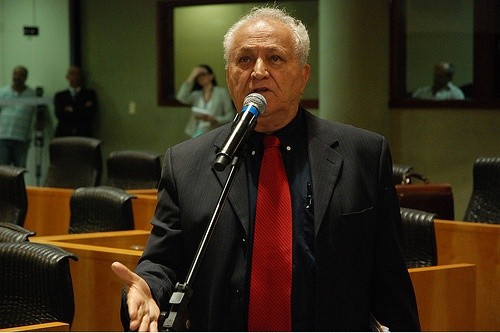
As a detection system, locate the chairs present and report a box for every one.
[105,150,162,189]
[68,184,139,235]
[394,162,429,187]
[47,136,103,188]
[0,165,30,226]
[0,242,79,329]
[400,207,440,269]
[464,155,500,223]
[0,221,36,243]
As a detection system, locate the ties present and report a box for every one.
[248,135,292,332]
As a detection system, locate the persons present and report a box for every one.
[111,7,421,333]
[54,66,103,187]
[176,65,234,138]
[412,61,464,100]
[0,66,37,169]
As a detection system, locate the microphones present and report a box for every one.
[214,93,267,171]
